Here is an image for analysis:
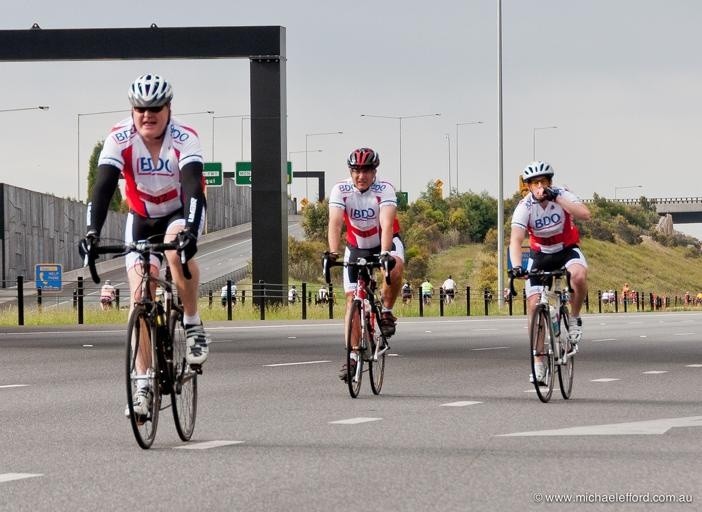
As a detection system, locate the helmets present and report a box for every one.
[128,73,173,107]
[348,149,379,169]
[522,161,554,183]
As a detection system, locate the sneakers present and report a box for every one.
[568,316,582,344]
[380,312,395,337]
[124,387,152,420]
[181,320,210,364]
[529,365,546,386]
[338,359,357,380]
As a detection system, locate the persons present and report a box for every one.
[326,146,406,382]
[98,276,701,315]
[82,72,211,420]
[508,161,592,386]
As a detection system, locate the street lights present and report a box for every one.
[443,133,452,194]
[288,149,323,199]
[613,184,643,199]
[170,110,214,116]
[361,113,440,208]
[0,105,50,112]
[455,120,483,197]
[305,131,343,206]
[532,125,558,161]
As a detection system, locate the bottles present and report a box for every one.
[151,287,167,328]
[363,299,372,320]
[549,306,560,337]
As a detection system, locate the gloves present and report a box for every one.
[322,252,341,268]
[379,251,396,271]
[78,228,100,260]
[171,227,197,262]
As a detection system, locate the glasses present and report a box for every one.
[134,106,162,113]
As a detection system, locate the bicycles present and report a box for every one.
[507,267,582,403]
[320,248,394,398]
[80,230,203,450]
